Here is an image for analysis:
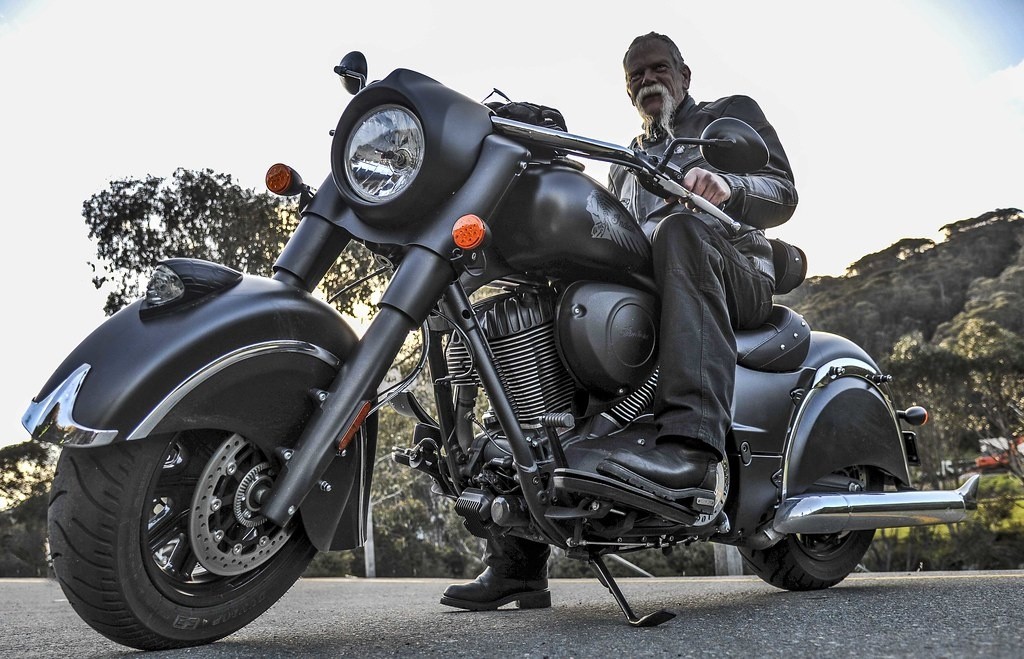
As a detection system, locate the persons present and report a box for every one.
[439,31,799,611]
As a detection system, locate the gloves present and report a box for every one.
[500,98,567,158]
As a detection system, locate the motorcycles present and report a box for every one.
[21,51,982,650]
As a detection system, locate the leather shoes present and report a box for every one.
[439,564,552,611]
[597,434,720,515]
